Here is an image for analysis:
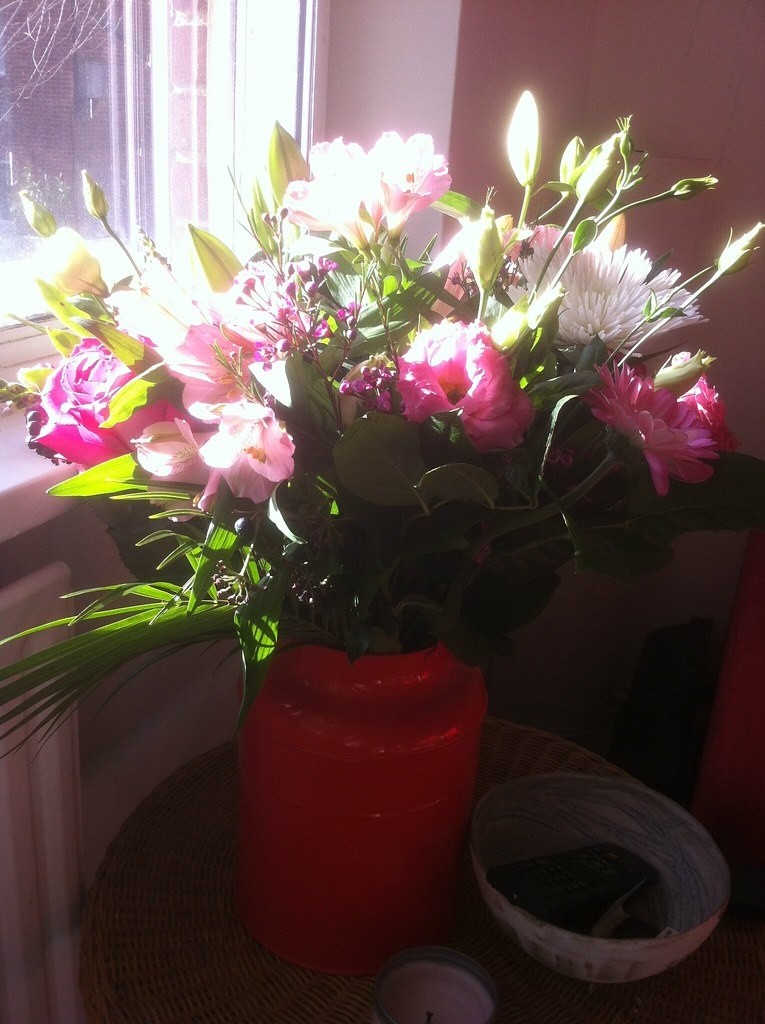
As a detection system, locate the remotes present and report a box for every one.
[486,842,643,921]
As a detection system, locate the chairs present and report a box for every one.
[480,528,749,803]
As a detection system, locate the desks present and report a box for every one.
[79,711,765,1024]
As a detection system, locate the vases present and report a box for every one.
[238,635,490,979]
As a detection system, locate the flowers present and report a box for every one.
[0,87,765,771]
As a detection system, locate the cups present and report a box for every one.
[373,944,498,1024]
